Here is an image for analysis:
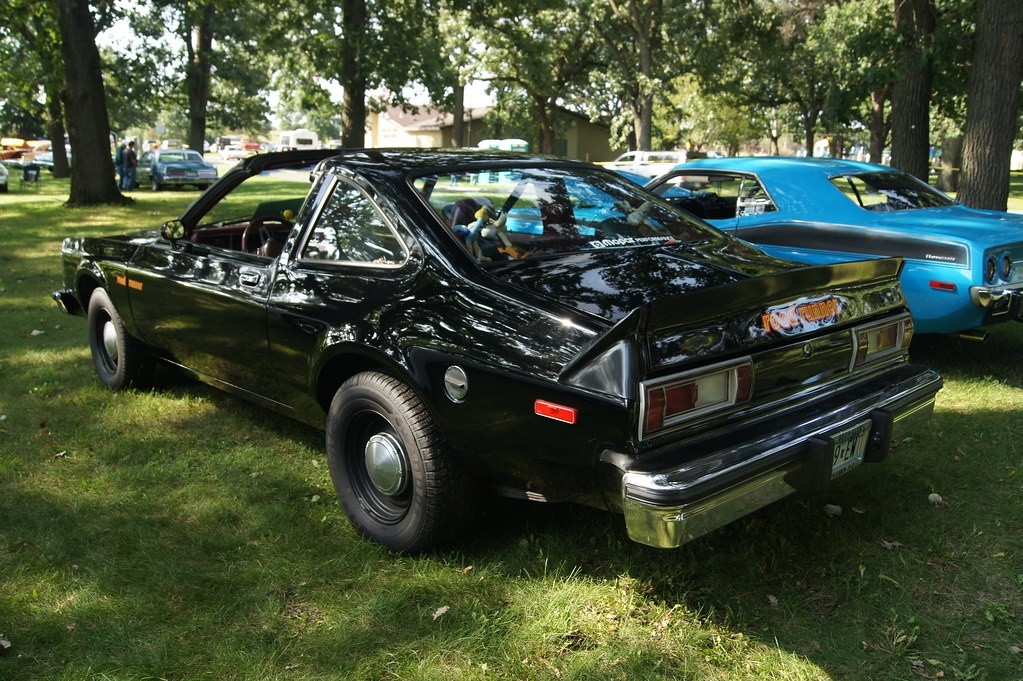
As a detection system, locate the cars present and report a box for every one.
[496,157,1022,333]
[50,148,943,555]
[277,129,318,151]
[225,145,244,160]
[241,138,259,149]
[602,151,709,191]
[135,149,218,192]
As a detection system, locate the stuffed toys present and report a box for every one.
[453,205,544,262]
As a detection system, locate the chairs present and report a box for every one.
[19,165,41,191]
[450,195,508,261]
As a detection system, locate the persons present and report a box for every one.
[115,141,139,190]
[450,175,457,186]
